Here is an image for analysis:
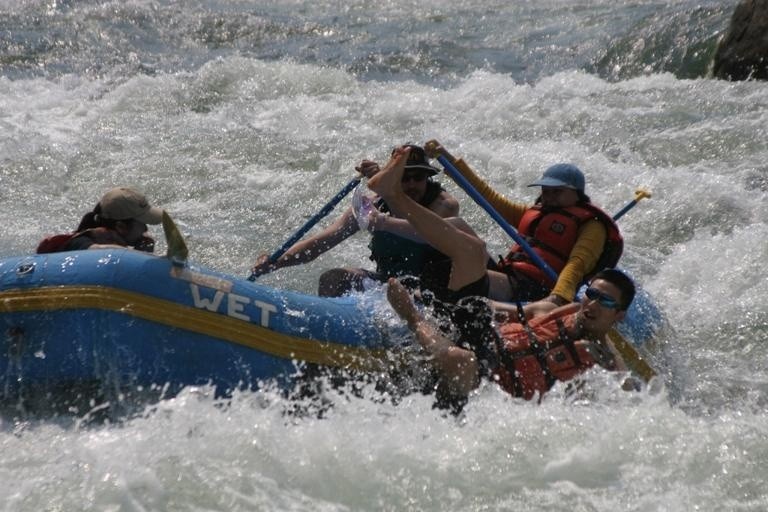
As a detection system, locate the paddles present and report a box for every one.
[160,209,190,262]
[429,142,656,392]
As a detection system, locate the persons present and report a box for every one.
[351,139,624,303]
[252,145,458,290]
[39,186,188,265]
[384,270,636,417]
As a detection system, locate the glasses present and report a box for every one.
[397,170,431,184]
[585,285,628,313]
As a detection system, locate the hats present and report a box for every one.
[96,187,164,226]
[386,145,441,178]
[527,163,586,196]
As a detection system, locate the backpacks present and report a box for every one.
[36,233,73,254]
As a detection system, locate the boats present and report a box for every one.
[0,249,668,410]
